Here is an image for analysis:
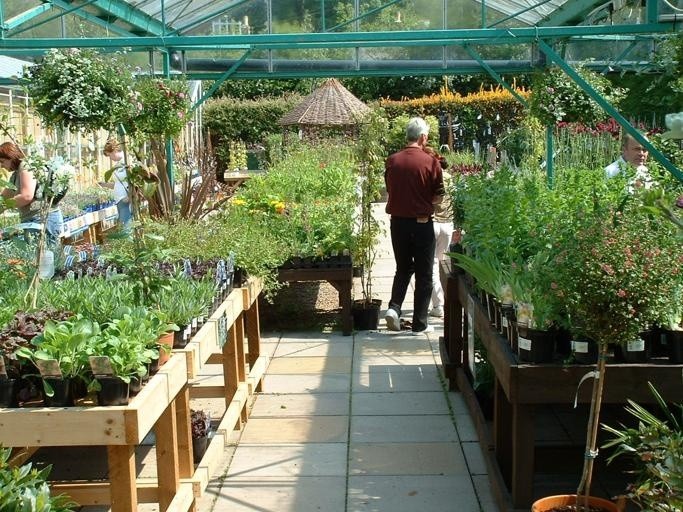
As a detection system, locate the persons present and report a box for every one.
[96,139,133,235]
[0,142,63,251]
[603,131,665,208]
[384,117,445,331]
[422,148,457,318]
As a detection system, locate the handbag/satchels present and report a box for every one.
[43,171,68,204]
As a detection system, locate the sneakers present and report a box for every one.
[412,325,435,336]
[429,307,444,317]
[385,309,400,330]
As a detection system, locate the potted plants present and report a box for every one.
[442,149,683,512]
[0,111,386,465]
